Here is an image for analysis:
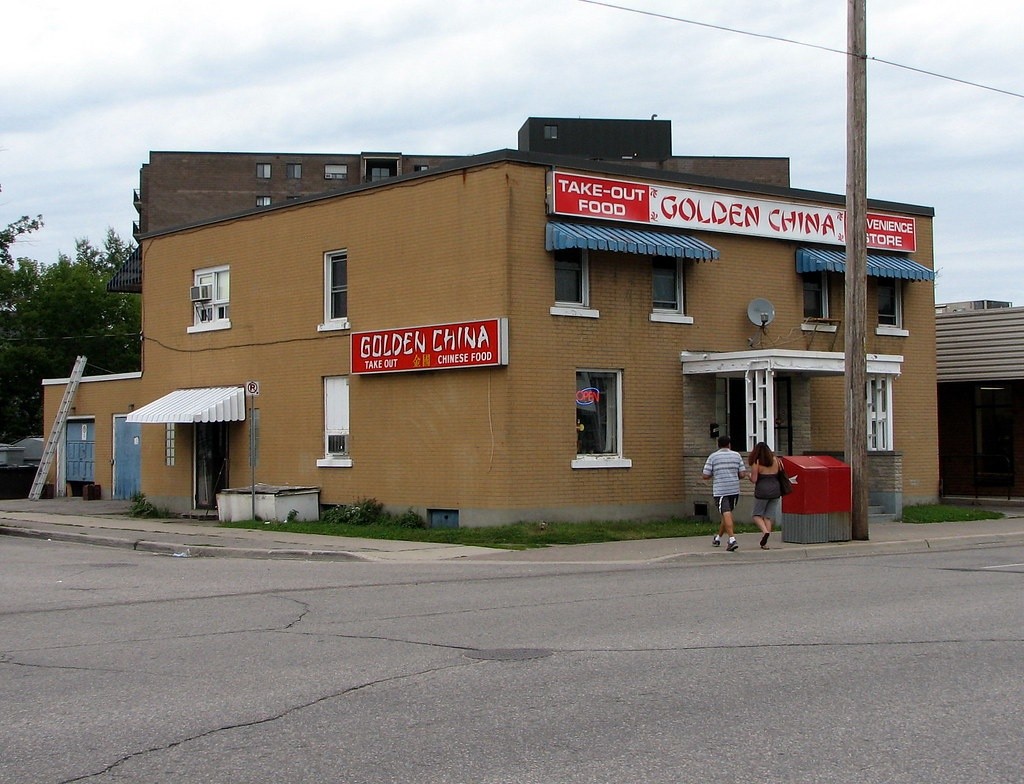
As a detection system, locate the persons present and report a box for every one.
[703,436,746,550]
[748,442,784,550]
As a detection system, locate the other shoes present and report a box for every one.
[760,533,769,546]
[761,544,770,550]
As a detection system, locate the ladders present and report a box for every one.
[27,353,87,501]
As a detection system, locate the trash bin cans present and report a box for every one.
[778,454,851,544]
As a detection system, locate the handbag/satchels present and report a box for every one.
[776,457,792,496]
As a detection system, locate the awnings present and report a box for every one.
[795,246,935,283]
[545,221,719,264]
[125,386,245,424]
[106,243,141,292]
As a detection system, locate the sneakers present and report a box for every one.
[712,536,720,547]
[726,540,738,551]
